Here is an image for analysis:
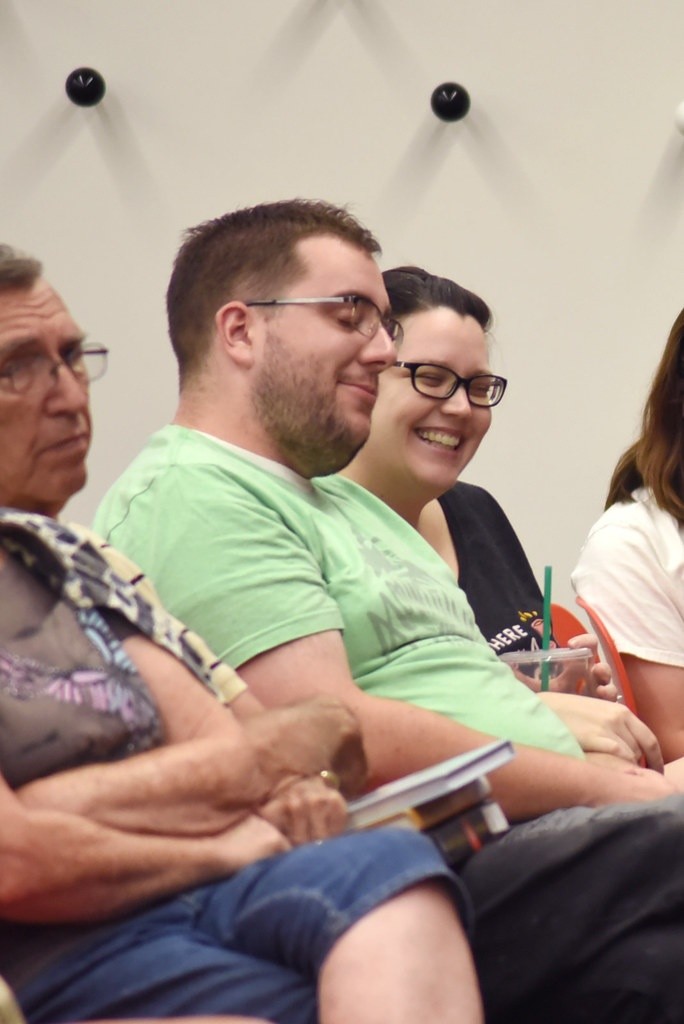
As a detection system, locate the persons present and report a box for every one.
[0,199,684,1024]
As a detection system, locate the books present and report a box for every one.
[368,776,493,835]
[428,805,512,872]
[346,740,517,830]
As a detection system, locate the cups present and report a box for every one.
[497,646,592,696]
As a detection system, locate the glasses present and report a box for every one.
[0,342,109,394]
[392,361,507,407]
[243,294,405,353]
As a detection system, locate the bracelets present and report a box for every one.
[320,768,340,788]
